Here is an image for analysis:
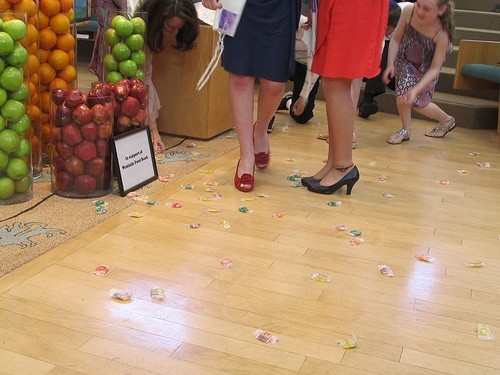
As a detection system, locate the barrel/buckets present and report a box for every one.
[0,0,149,206]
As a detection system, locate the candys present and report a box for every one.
[93,134,493,346]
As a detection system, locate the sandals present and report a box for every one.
[387,128,410,144]
[267,115,276,131]
[426,116,455,137]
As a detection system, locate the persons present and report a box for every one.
[202,0,303,192]
[268,0,456,145]
[91,0,200,154]
[300,0,390,195]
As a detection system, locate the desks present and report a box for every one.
[152,22,234,140]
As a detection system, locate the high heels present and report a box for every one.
[307,166,360,195]
[301,176,321,187]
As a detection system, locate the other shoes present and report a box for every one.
[278,91,293,110]
[359,99,378,118]
[233,157,254,192]
[252,120,269,169]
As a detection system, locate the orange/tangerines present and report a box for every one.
[0,0,76,166]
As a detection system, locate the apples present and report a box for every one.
[104,15,146,85]
[48,79,148,195]
[0,20,30,200]
